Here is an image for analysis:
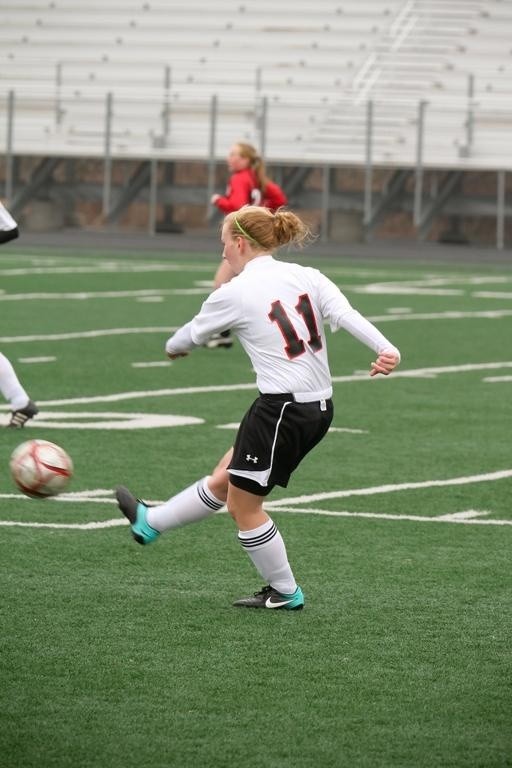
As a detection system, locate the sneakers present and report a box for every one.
[232,584,305,610]
[203,333,233,348]
[7,400,39,430]
[116,484,161,546]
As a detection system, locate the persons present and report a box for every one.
[0,202,38,429]
[115,205,402,610]
[202,141,288,348]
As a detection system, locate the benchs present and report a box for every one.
[0,1,511,169]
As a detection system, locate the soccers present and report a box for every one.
[11,438,74,498]
